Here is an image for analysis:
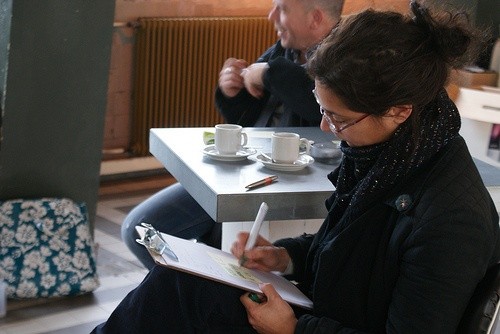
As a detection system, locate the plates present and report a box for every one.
[255,153,315,171]
[202,145,257,161]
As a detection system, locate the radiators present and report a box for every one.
[127,14,280,158]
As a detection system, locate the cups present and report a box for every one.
[214,124,247,155]
[271,133,311,163]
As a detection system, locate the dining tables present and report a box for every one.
[148,123,342,256]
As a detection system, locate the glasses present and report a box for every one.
[312,88,372,136]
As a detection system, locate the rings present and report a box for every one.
[242,67,249,74]
[224,68,232,75]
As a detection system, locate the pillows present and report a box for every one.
[0,197,99,300]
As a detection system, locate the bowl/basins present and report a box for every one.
[311,140,342,164]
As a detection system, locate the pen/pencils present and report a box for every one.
[241,202,268,264]
[244,175,277,190]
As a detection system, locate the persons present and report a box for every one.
[121,0,346,272]
[91,0,500,334]
